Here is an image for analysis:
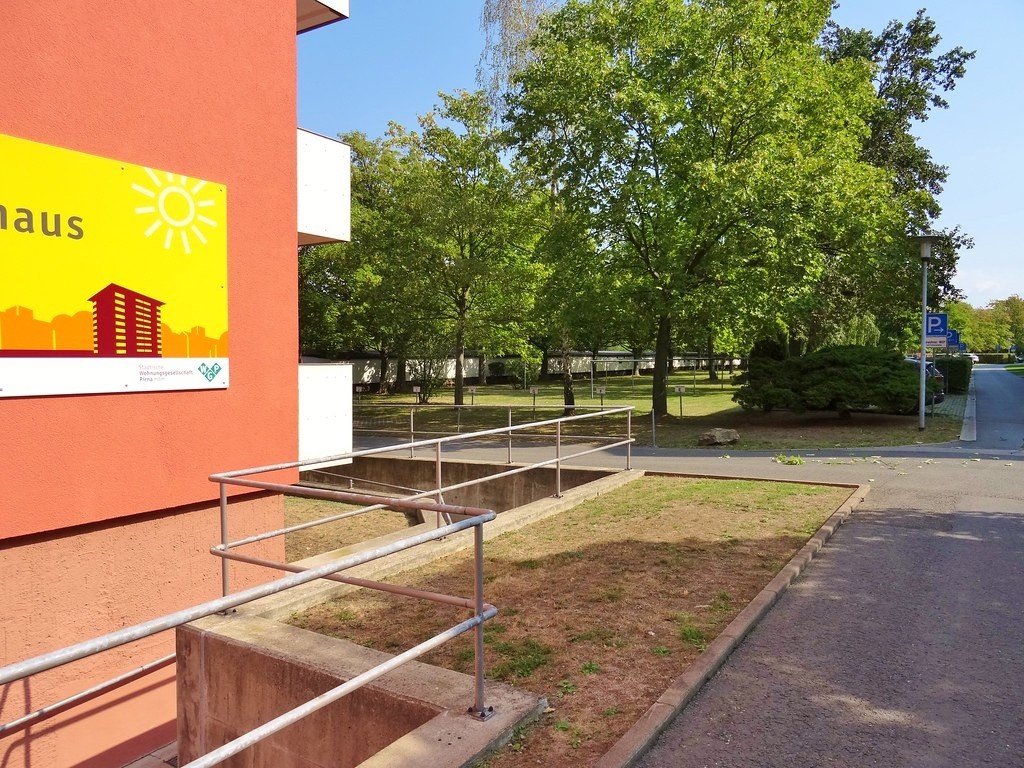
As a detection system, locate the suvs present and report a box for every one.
[904,359,945,406]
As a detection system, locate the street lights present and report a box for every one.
[912,235,945,430]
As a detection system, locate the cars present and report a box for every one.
[963,353,979,364]
